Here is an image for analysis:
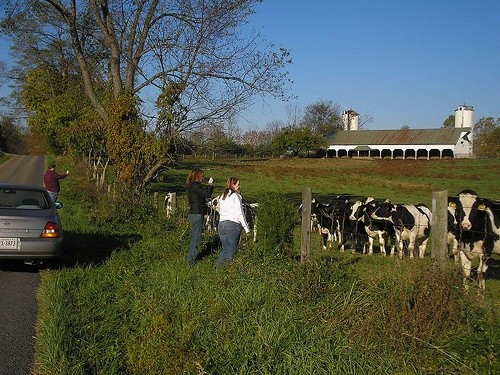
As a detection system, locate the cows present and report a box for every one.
[449,188,500,293]
[298,197,432,259]
[165,194,259,242]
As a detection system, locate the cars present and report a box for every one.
[0,182,65,261]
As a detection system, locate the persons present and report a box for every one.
[44,164,70,204]
[185,168,214,266]
[207,177,251,268]
